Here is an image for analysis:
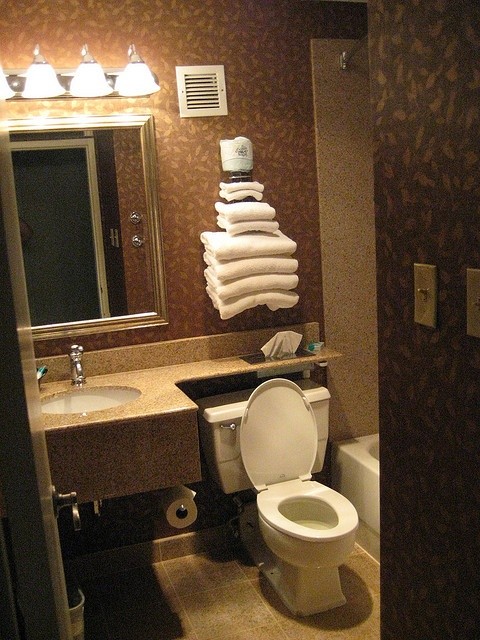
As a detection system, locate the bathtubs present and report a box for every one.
[327,429,381,565]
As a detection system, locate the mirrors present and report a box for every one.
[7,114,169,343]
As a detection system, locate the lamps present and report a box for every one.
[0,67,16,100]
[21,45,67,98]
[71,44,114,98]
[114,44,160,98]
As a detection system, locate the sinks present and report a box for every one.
[35,383,142,416]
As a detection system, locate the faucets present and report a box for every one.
[67,346,86,387]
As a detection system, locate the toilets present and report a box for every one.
[196,377,357,618]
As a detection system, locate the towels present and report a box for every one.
[219,181,264,193]
[200,227,297,259]
[217,216,279,236]
[207,273,299,301]
[218,190,263,201]
[205,286,299,320]
[220,137,254,172]
[214,202,276,222]
[203,256,299,281]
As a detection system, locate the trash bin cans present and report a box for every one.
[65,582,86,640]
